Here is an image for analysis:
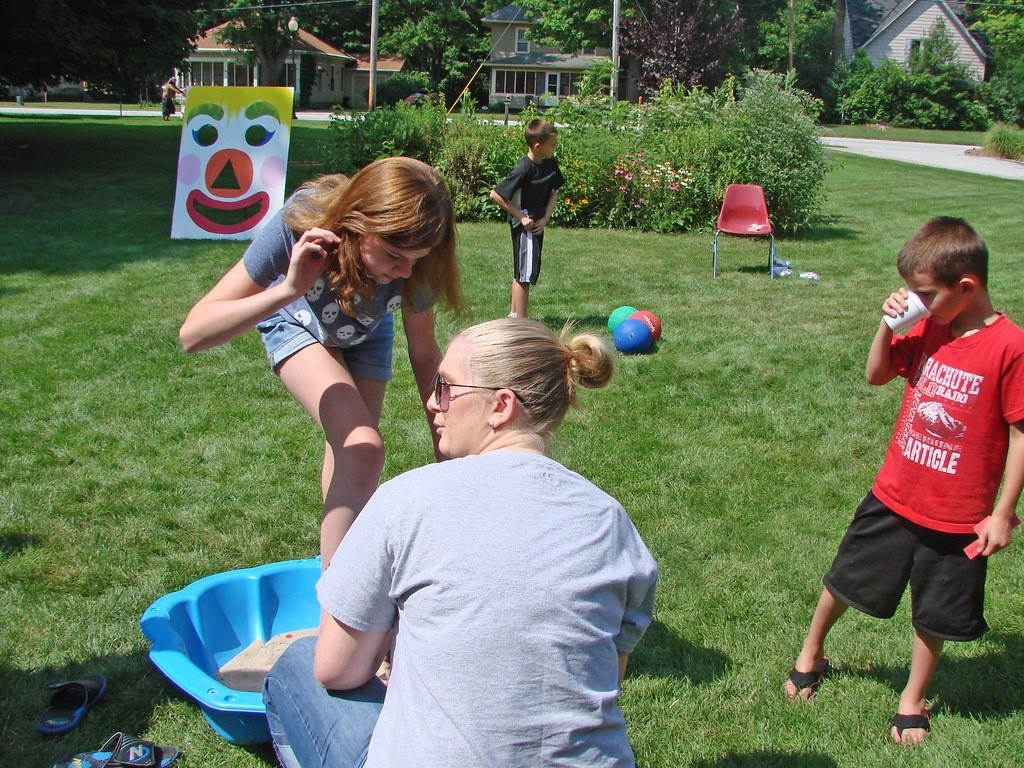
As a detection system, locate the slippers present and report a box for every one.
[38,675,106,733]
[52,731,185,768]
[784,656,829,702]
[887,709,933,747]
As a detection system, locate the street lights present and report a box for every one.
[289,15,300,120]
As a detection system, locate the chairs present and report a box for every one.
[712,184,774,276]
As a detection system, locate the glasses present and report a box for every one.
[434,373,532,409]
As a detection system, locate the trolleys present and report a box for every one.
[181,90,186,119]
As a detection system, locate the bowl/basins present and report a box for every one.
[142,560,332,747]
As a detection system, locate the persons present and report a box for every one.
[179,156,477,575]
[160,77,186,122]
[785,215,1024,745]
[262,317,658,768]
[489,119,567,317]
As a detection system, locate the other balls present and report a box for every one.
[607,305,662,354]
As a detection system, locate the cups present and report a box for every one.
[884,290,930,332]
